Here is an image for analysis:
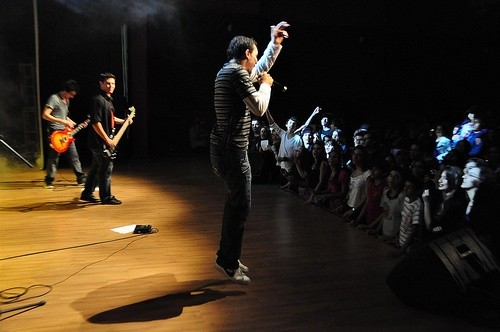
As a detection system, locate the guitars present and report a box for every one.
[47,118,90,154]
[99,106,136,162]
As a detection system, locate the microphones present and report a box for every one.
[257,72,287,91]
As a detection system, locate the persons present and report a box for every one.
[210,21,290,285]
[252,106,500,256]
[43,79,86,188]
[78,74,133,204]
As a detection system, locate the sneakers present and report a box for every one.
[215,261,251,284]
[215,254,249,273]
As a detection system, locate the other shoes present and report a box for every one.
[78,177,87,184]
[101,196,121,205]
[43,178,54,189]
[80,194,101,203]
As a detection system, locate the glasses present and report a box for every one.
[466,171,482,180]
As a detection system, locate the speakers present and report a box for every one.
[387,224,500,316]
